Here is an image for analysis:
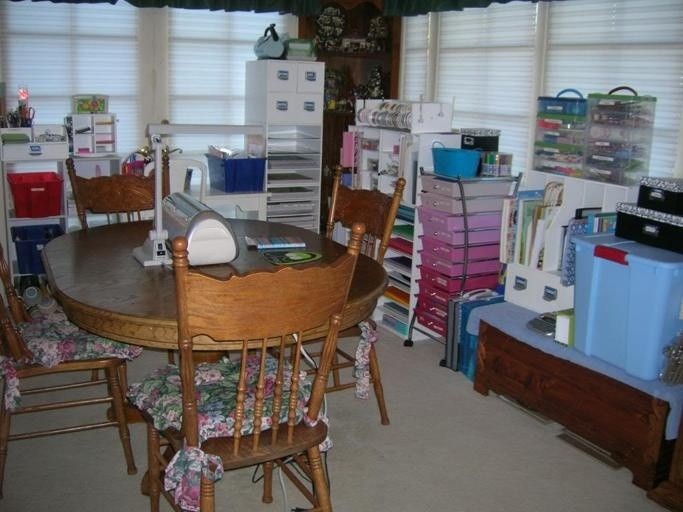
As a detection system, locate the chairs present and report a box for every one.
[292,171,403,439]
[147,222,365,510]
[0,244,141,479]
[64,155,175,226]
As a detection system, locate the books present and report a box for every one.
[266,127,317,230]
[245,234,307,251]
[378,204,415,338]
[498,185,579,280]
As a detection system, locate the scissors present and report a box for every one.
[7,107,34,127]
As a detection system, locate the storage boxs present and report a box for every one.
[532,84,657,186]
[9,224,67,276]
[569,175,682,384]
[7,169,65,216]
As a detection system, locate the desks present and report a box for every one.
[40,219,392,501]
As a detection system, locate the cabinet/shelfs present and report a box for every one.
[503,169,633,313]
[340,97,522,368]
[475,302,668,490]
[147,58,326,236]
[0,143,123,278]
[63,112,118,159]
